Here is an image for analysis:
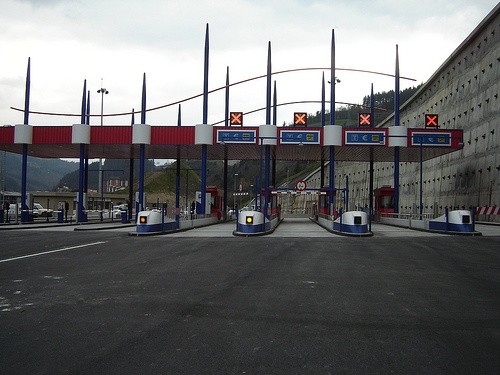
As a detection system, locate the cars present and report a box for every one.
[4,202,39,217]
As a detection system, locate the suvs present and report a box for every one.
[33,203,53,217]
